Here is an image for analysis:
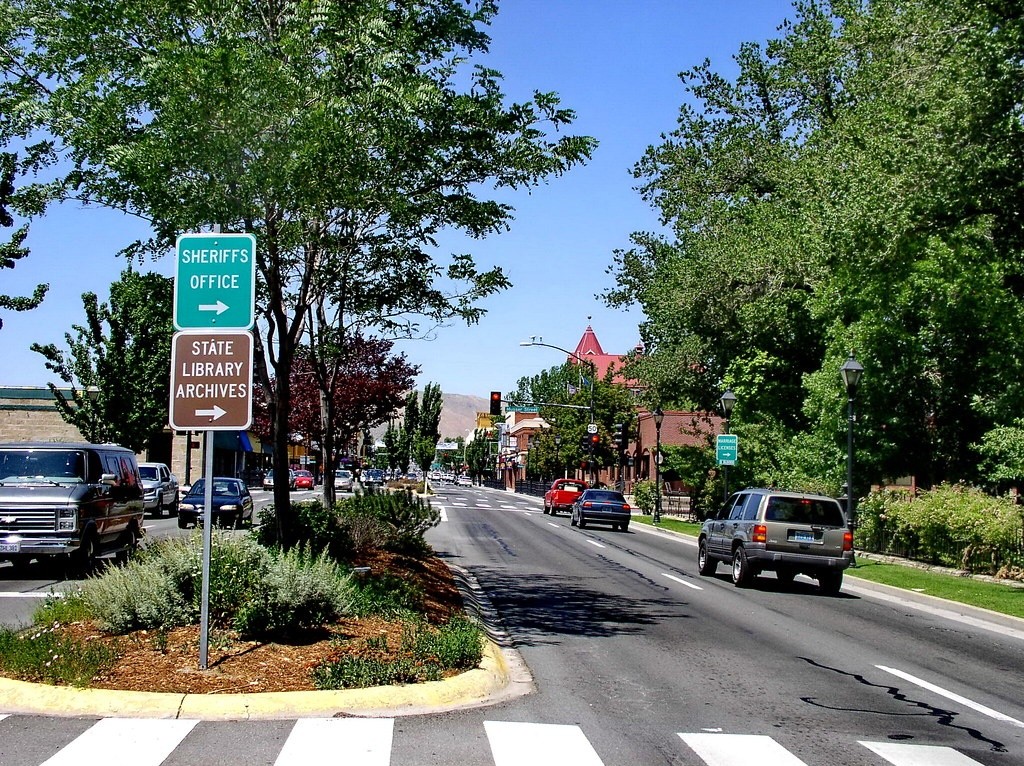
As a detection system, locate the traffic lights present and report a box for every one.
[190,441,200,449]
[614,423,628,450]
[588,433,600,449]
[489,391,501,415]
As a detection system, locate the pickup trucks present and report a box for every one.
[544,478,590,516]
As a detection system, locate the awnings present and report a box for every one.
[340,458,352,463]
[213,430,273,455]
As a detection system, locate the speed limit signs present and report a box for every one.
[587,423,598,434]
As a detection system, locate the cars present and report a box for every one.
[262,468,297,491]
[293,469,315,490]
[333,467,475,496]
[177,476,254,530]
[571,488,631,532]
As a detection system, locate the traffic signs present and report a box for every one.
[716,433,738,461]
[172,232,257,331]
[167,329,253,431]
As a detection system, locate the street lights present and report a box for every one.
[527,438,538,482]
[838,347,865,569]
[520,341,595,488]
[553,435,562,479]
[720,387,737,503]
[651,405,665,523]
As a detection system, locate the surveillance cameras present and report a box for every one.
[540,336,542,341]
[529,335,536,338]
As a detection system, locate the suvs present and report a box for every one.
[697,487,854,597]
[136,462,180,520]
[0,440,147,580]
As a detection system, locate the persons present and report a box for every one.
[223,483,238,495]
